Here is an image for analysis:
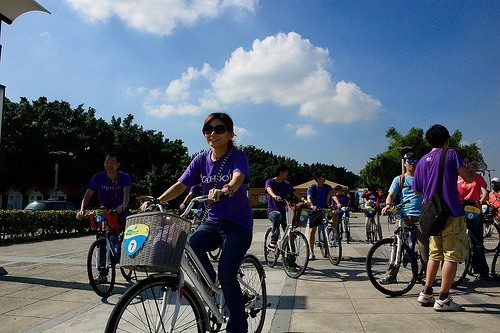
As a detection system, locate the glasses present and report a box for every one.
[321,175,326,178]
[407,160,419,165]
[203,124,226,134]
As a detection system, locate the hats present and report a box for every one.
[403,152,418,159]
[491,177,500,183]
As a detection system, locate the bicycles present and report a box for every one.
[263,198,310,279]
[440,200,500,292]
[183,202,223,260]
[104,191,271,333]
[362,206,378,244]
[341,207,352,243]
[366,200,426,297]
[316,206,345,266]
[79,210,135,298]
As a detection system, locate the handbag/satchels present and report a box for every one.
[419,194,448,236]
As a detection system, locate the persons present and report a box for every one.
[380,151,437,285]
[141,112,253,333]
[333,185,351,240]
[307,172,342,259]
[458,155,500,281]
[77,152,131,284]
[265,165,309,270]
[412,124,473,311]
[363,186,383,241]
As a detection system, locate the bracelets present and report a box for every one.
[224,184,234,197]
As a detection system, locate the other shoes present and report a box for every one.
[268,241,277,251]
[417,290,435,304]
[433,295,462,311]
[379,275,397,285]
[94,275,108,284]
[309,253,315,261]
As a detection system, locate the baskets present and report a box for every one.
[285,205,311,228]
[120,211,192,273]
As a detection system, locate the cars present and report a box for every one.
[24,201,74,213]
